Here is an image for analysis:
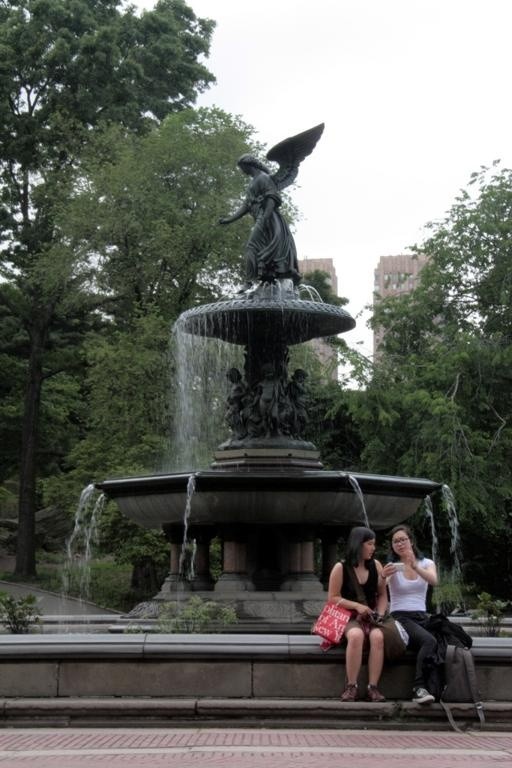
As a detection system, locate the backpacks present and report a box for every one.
[439,645,486,732]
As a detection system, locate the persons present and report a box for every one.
[328,526,388,702]
[378,524,448,705]
[224,365,316,440]
[219,122,326,288]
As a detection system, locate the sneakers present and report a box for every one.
[341,683,360,702]
[411,687,435,704]
[367,684,385,702]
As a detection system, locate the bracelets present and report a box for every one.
[412,565,418,570]
[380,571,386,579]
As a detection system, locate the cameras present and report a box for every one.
[393,563,405,573]
[372,613,377,620]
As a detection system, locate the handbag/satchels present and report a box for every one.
[376,613,408,657]
[311,602,352,644]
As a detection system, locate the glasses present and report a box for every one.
[392,537,409,545]
